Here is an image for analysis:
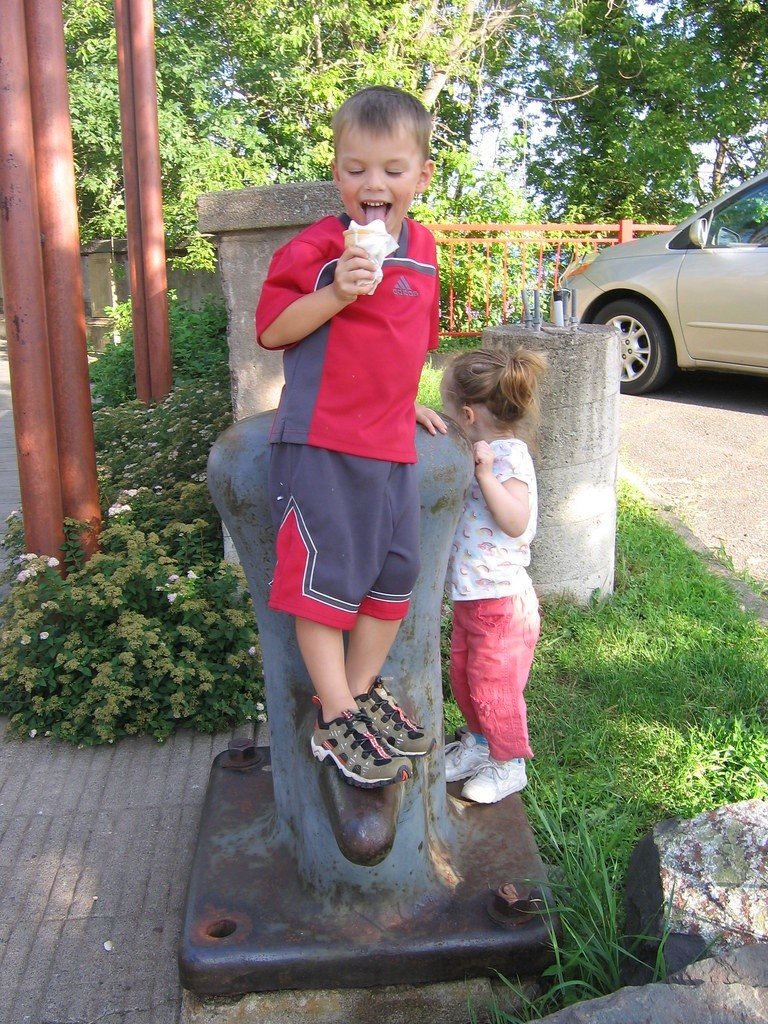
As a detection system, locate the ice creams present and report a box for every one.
[342,218,387,250]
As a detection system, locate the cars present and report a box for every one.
[557,171,767,397]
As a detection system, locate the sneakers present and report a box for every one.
[311,696,413,790]
[352,674,438,756]
[462,757,527,803]
[444,731,490,782]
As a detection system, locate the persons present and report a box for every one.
[253,85,449,788]
[437,346,544,805]
